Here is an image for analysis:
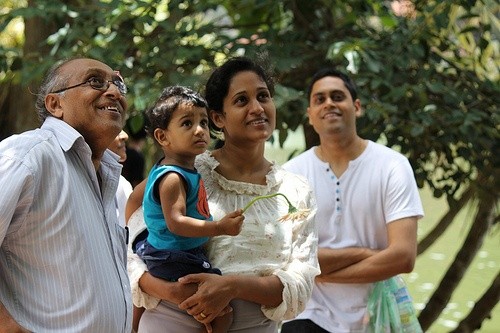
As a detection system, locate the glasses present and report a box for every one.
[50,76,127,94]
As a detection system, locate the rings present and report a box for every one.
[200,313,206,318]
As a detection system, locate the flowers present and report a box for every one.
[241,193,312,225]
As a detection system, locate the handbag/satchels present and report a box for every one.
[362,277,422,333]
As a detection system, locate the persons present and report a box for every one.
[281,70,424,333]
[125,85,245,333]
[103,130,133,232]
[127,56,321,333]
[0,55,146,333]
